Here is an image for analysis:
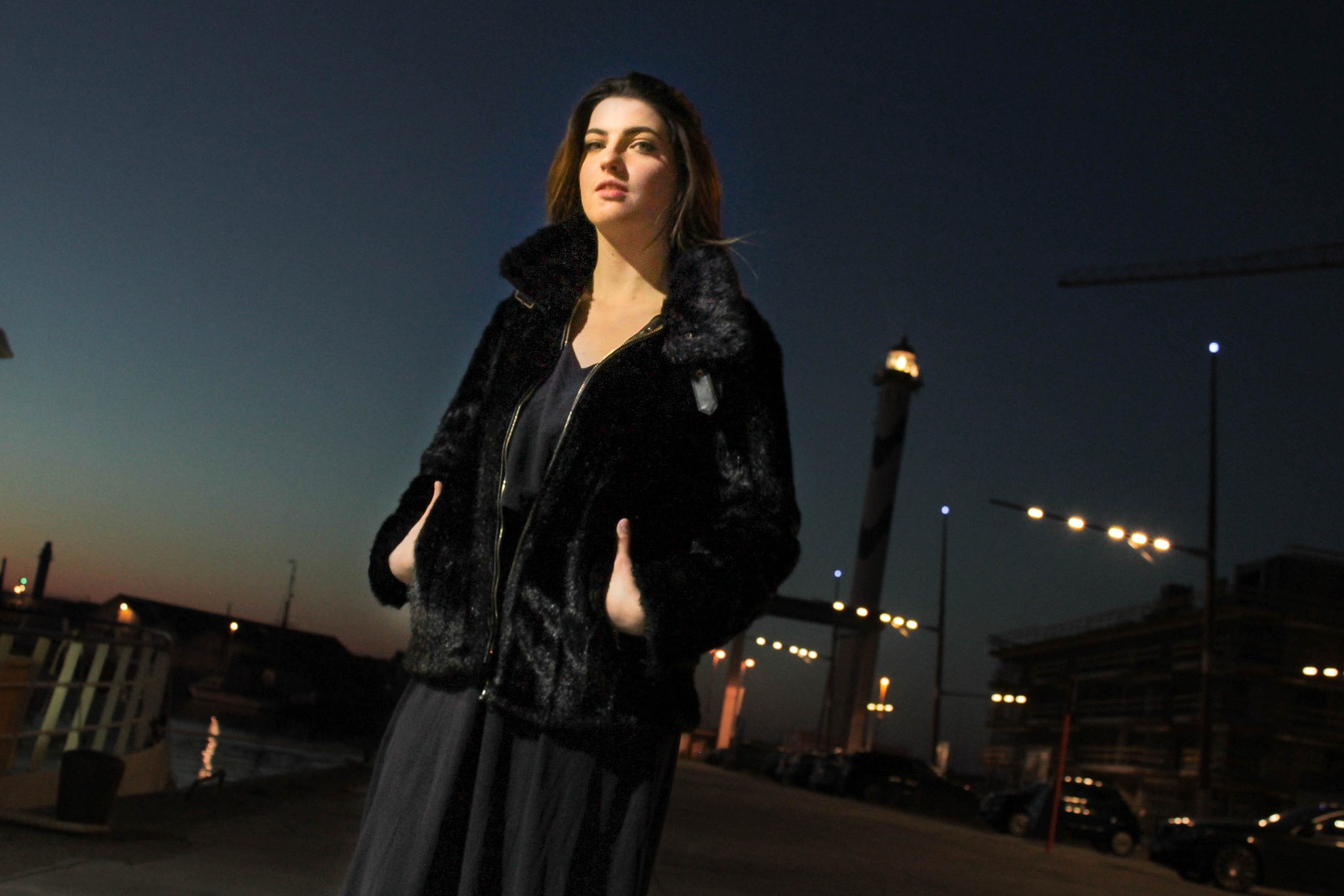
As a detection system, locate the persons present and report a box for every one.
[338,67,805,896]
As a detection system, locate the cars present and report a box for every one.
[975,776,1144,858]
[1149,808,1344,896]
[705,742,979,826]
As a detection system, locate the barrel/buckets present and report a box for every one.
[55,748,126,825]
